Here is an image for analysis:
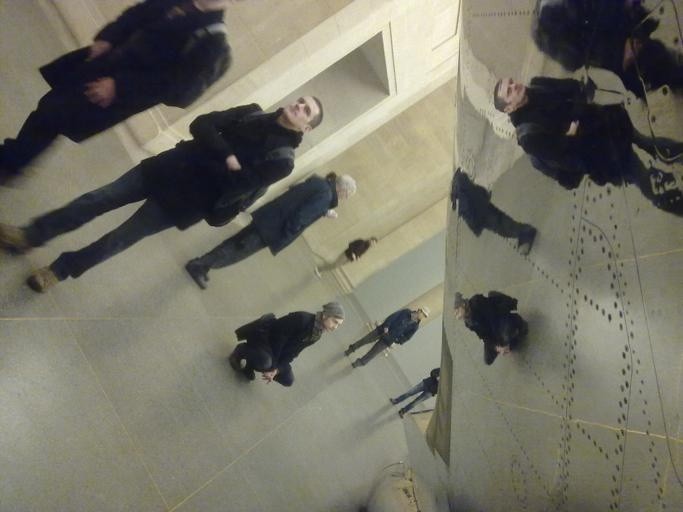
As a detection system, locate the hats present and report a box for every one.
[420,307,429,316]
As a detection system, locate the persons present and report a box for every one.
[0,0,234,189]
[346,304,431,369]
[312,235,377,279]
[492,74,683,212]
[229,300,345,390]
[184,170,358,292]
[452,289,529,366]
[0,88,325,296]
[449,166,537,256]
[390,367,441,420]
[528,0,683,98]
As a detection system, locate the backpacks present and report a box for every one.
[162,22,232,109]
[514,82,583,189]
[205,111,296,227]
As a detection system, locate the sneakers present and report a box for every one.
[513,226,542,346]
[0,222,31,254]
[229,265,406,417]
[29,265,59,294]
[185,259,209,289]
